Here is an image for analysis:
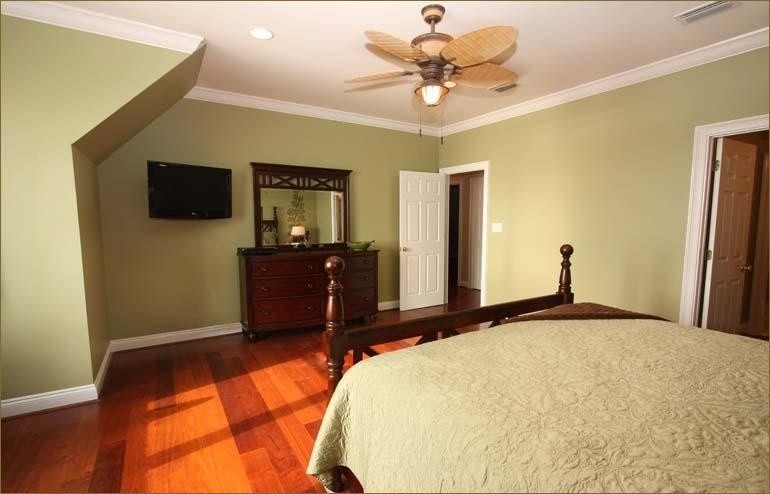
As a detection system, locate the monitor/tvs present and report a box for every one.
[147,160,232,219]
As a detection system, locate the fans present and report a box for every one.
[343,5,520,91]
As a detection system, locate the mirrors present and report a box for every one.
[250,161,353,250]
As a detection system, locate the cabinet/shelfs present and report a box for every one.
[237,247,381,343]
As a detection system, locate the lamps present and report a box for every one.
[414,79,449,106]
[290,226,306,243]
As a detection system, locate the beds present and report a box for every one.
[324,244,770,494]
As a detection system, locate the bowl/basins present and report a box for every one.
[346,241,371,252]
